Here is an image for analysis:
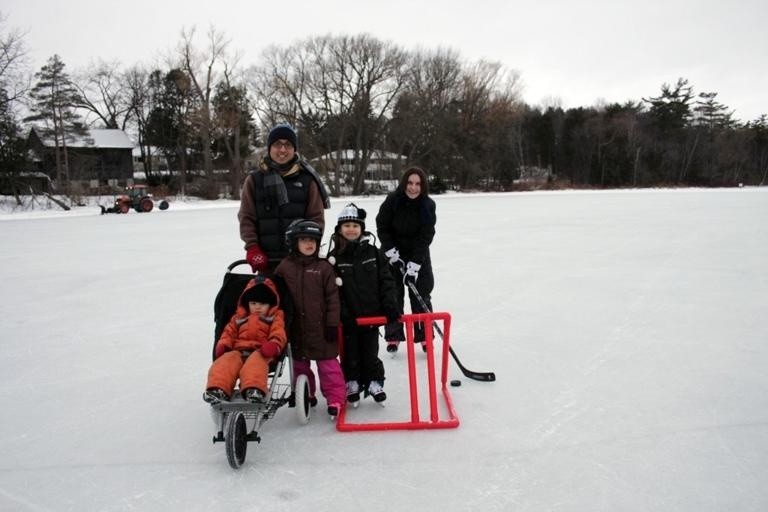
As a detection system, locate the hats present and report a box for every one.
[268,127,297,152]
[338,203,366,231]
[285,218,321,253]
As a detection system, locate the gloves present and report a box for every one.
[215,344,225,358]
[380,303,401,325]
[323,327,338,343]
[384,246,405,269]
[261,342,277,357]
[403,261,421,284]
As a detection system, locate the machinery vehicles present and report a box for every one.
[114,185,153,213]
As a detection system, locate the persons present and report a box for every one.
[202,275,287,402]
[375,167,436,352]
[237,123,328,376]
[271,216,347,415]
[327,201,401,401]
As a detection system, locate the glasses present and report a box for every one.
[272,140,293,149]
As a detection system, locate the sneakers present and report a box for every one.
[245,388,263,402]
[387,341,399,352]
[327,380,360,416]
[202,388,228,403]
[247,246,268,274]
[365,380,386,402]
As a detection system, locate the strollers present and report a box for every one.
[209,256,312,467]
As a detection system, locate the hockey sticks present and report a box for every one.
[398,266,496,382]
